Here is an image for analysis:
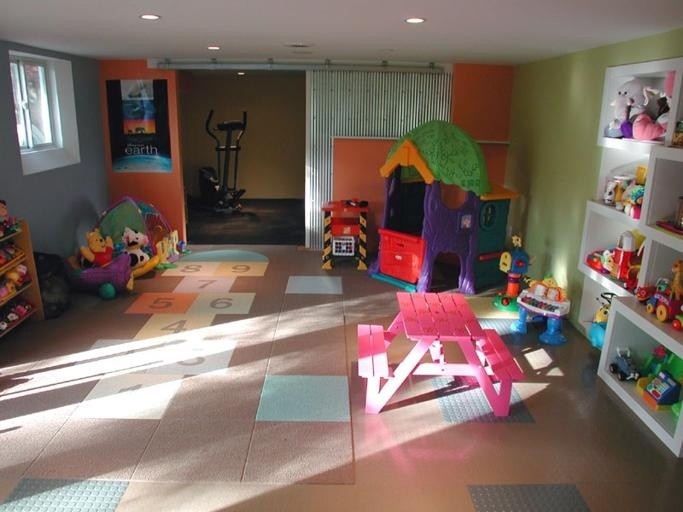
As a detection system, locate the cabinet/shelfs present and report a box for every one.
[578,57,683,459]
[0,219,45,337]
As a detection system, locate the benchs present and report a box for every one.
[476,329,525,417]
[357,324,389,414]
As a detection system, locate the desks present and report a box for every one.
[378,291,498,417]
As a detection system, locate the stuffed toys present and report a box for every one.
[1,262,31,300]
[603,163,649,222]
[0,242,25,267]
[0,198,23,237]
[632,69,675,142]
[604,76,659,138]
[80,228,114,267]
[0,295,34,332]
[121,227,150,271]
